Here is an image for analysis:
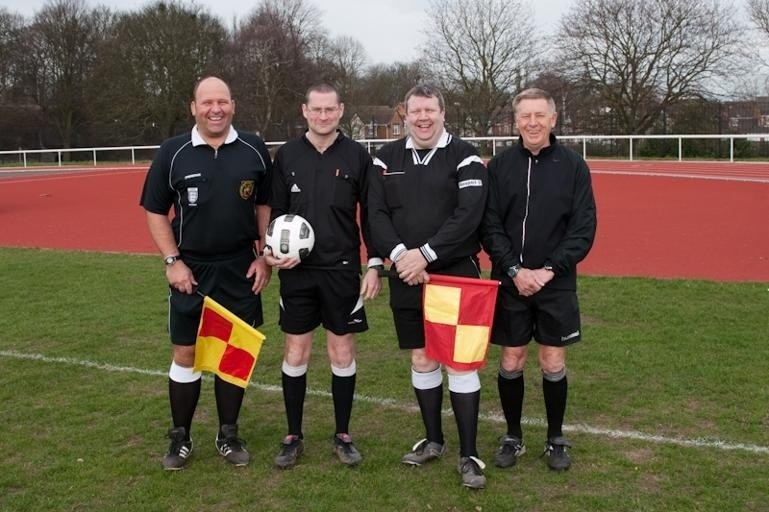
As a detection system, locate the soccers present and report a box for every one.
[266,214,315,263]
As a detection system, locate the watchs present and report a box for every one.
[164,255,181,266]
[545,263,557,275]
[507,263,520,278]
[368,264,385,278]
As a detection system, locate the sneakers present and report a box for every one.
[401,439,446,466]
[332,433,362,465]
[275,435,304,467]
[457,456,487,489]
[162,427,193,471]
[215,424,249,466]
[539,439,572,469]
[495,434,526,468]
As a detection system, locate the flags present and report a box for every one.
[193,294,266,390]
[422,274,499,372]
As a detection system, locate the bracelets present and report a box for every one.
[259,250,264,257]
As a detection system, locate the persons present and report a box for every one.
[262,82,385,470]
[140,77,273,471]
[368,83,488,490]
[478,87,598,473]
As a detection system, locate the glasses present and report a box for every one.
[306,106,340,113]
